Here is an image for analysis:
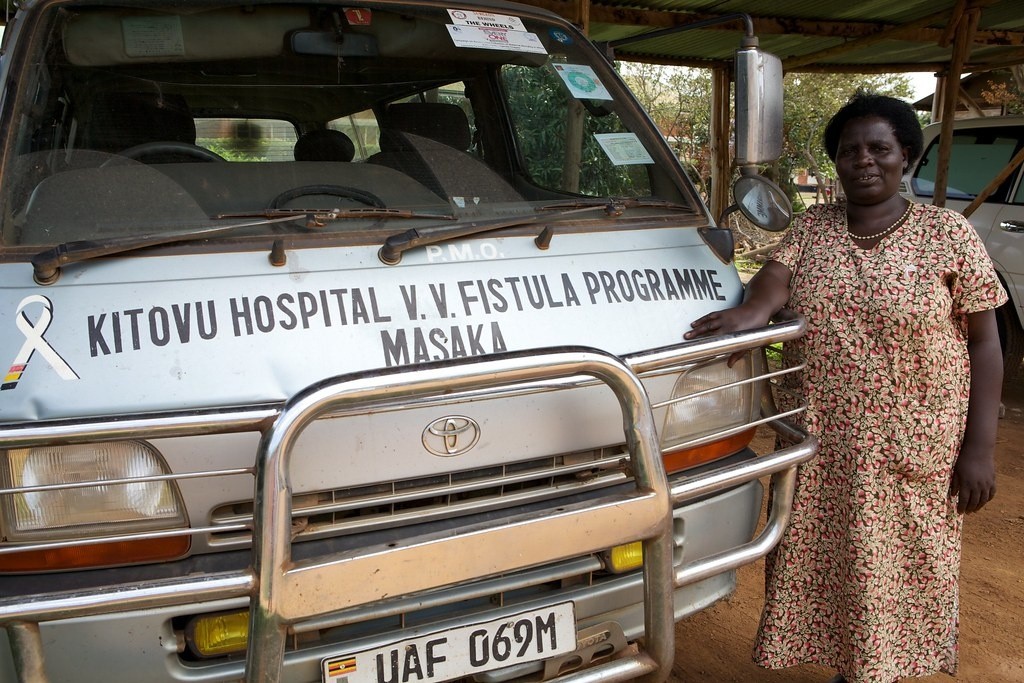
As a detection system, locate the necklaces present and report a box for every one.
[849,198,912,240]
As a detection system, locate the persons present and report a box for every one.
[682,85,1010,683]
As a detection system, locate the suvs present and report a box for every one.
[898,117,1024,392]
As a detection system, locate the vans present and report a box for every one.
[0,0,820,683]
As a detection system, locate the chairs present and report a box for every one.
[90,94,208,163]
[294,131,356,163]
[364,103,488,166]
